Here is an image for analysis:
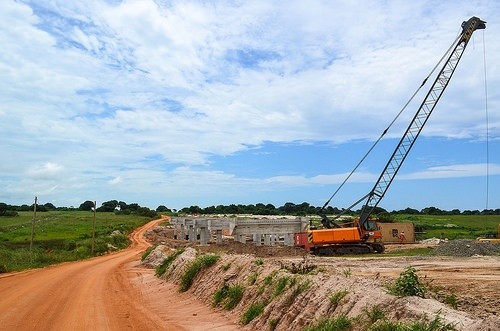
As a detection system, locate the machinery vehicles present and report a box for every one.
[298,16,487,257]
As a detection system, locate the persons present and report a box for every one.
[321,214,330,229]
